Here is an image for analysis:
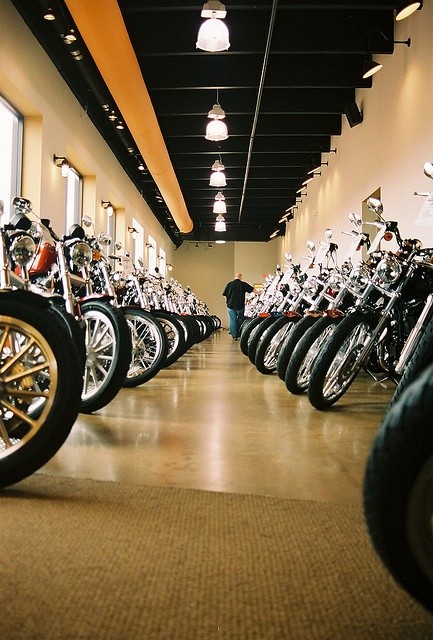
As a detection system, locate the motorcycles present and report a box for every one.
[276,228,345,385]
[387,164,432,427]
[1,186,87,492]
[84,217,165,385]
[155,265,220,369]
[41,185,131,416]
[285,224,384,400]
[235,268,280,375]
[256,240,323,377]
[365,394,432,622]
[309,200,431,409]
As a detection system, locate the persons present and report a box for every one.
[220,272,264,341]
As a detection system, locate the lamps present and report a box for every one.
[44,9,56,21]
[128,225,139,240]
[195,243,199,248]
[279,200,302,223]
[269,224,281,240]
[213,192,227,213]
[361,38,411,79]
[166,263,174,272]
[195,1,231,52]
[296,172,322,194]
[53,154,71,178]
[101,200,113,217]
[138,165,144,171]
[65,30,77,42]
[116,122,124,130]
[109,111,117,121]
[157,255,164,261]
[204,103,229,141]
[208,160,227,187]
[146,243,154,253]
[209,243,213,249]
[215,214,227,232]
[301,161,329,185]
[307,148,337,175]
[395,0,421,22]
